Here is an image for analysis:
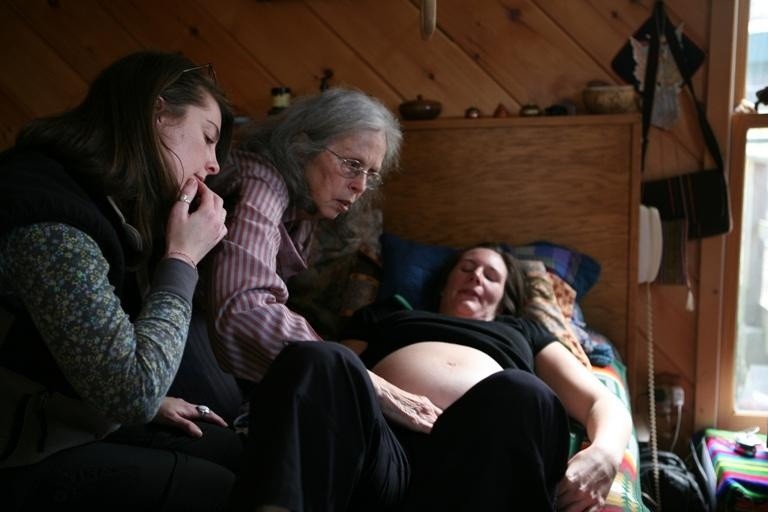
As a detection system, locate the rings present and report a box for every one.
[195,404,211,420]
[175,190,196,206]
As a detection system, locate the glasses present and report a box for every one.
[323,145,383,192]
[183,62,218,84]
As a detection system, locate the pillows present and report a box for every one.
[372,236,603,350]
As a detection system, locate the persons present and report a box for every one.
[240,240,633,508]
[191,83,445,435]
[0,49,249,505]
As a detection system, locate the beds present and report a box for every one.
[241,107,642,512]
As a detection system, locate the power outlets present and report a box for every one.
[653,373,679,415]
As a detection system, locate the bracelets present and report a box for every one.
[161,247,200,270]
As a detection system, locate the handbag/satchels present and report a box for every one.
[641,167,733,241]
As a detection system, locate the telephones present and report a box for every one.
[638,202,664,283]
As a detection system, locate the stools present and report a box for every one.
[702,427,766,512]
